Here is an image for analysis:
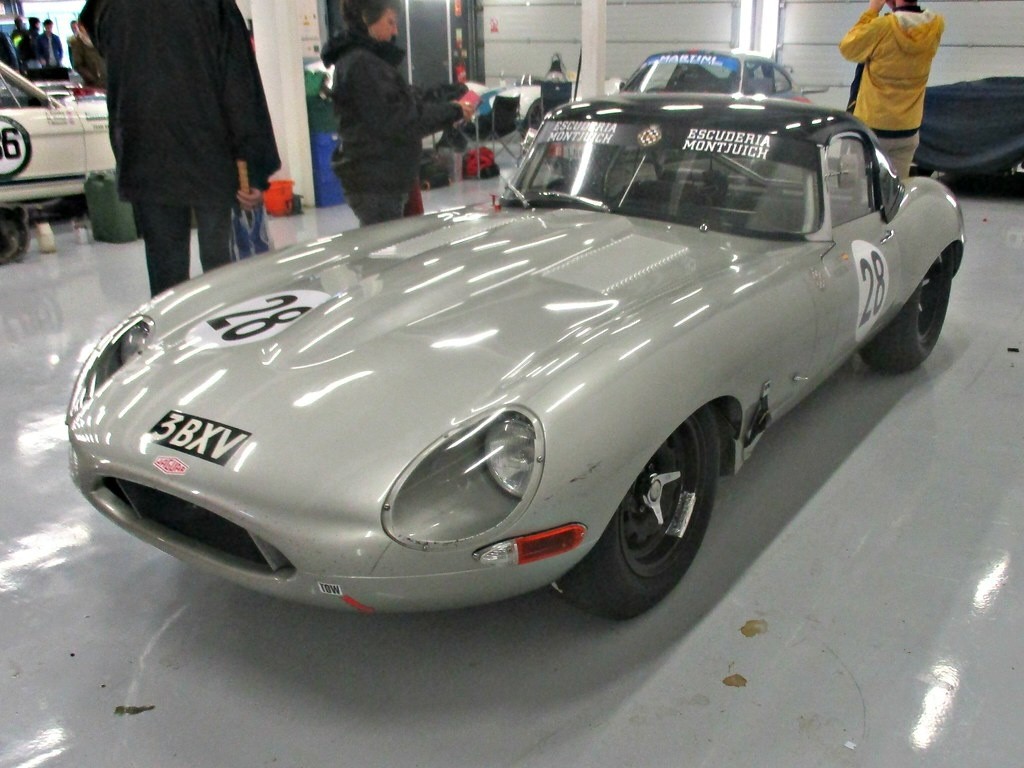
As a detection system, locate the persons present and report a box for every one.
[11,18,28,50]
[320,0,476,227]
[78,0,282,298]
[840,0,944,177]
[73,17,107,89]
[67,21,79,65]
[40,19,64,68]
[19,18,47,74]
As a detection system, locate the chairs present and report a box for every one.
[471,94,523,165]
[434,110,497,179]
[517,79,573,149]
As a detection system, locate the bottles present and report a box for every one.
[34,212,57,253]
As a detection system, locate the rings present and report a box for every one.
[465,102,469,106]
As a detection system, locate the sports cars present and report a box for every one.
[73,95,963,614]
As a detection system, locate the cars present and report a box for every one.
[635,52,788,95]
[3,60,150,256]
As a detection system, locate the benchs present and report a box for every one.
[619,181,865,231]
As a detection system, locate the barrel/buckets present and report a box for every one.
[83,167,139,244]
[261,180,296,218]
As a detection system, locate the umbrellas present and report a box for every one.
[229,159,274,263]
